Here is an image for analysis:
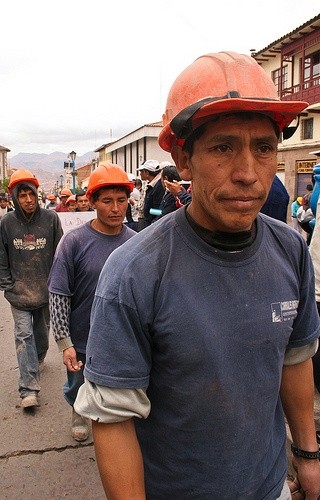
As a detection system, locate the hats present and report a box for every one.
[297,197,303,205]
[136,160,160,172]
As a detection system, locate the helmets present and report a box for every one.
[48,195,55,200]
[61,189,72,195]
[157,51,310,152]
[84,164,135,198]
[8,169,39,196]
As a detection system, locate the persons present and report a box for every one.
[73,52,320,500]
[122,160,192,233]
[46,161,138,442]
[0,168,65,407]
[0,188,95,218]
[307,194,320,393]
[303,184,313,203]
[291,196,304,234]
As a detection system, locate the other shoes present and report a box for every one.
[71,409,89,442]
[22,395,39,407]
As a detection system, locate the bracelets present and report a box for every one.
[291,443,320,459]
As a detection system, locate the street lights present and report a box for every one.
[69,150,77,195]
[60,175,63,192]
[54,179,59,197]
